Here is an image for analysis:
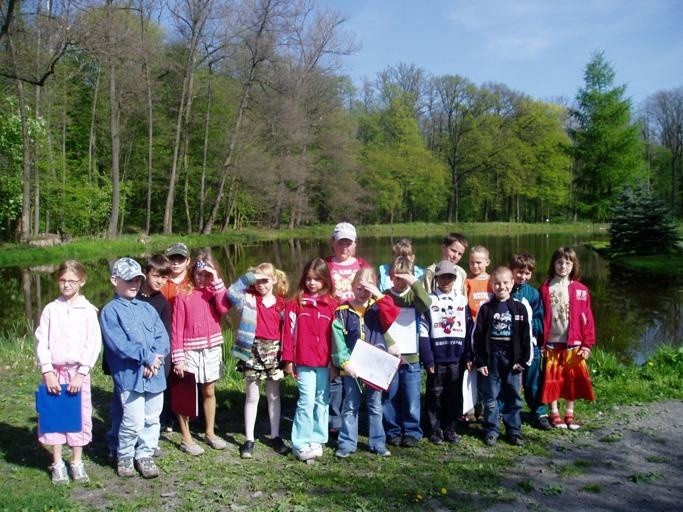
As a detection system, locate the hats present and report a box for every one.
[111,256,147,282]
[165,242,191,261]
[331,222,357,243]
[434,260,458,278]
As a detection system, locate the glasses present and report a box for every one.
[57,278,81,285]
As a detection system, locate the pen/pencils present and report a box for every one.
[355,377,363,393]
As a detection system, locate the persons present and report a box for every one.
[34,260,102,487]
[322,221,376,431]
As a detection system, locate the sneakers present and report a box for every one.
[294,443,323,461]
[48,461,70,487]
[204,433,227,450]
[334,449,352,458]
[509,436,524,446]
[69,460,90,486]
[179,441,206,456]
[484,432,499,447]
[387,435,417,448]
[536,414,581,431]
[267,437,291,455]
[116,457,136,478]
[134,456,160,479]
[240,440,255,459]
[369,447,392,457]
[428,427,461,446]
